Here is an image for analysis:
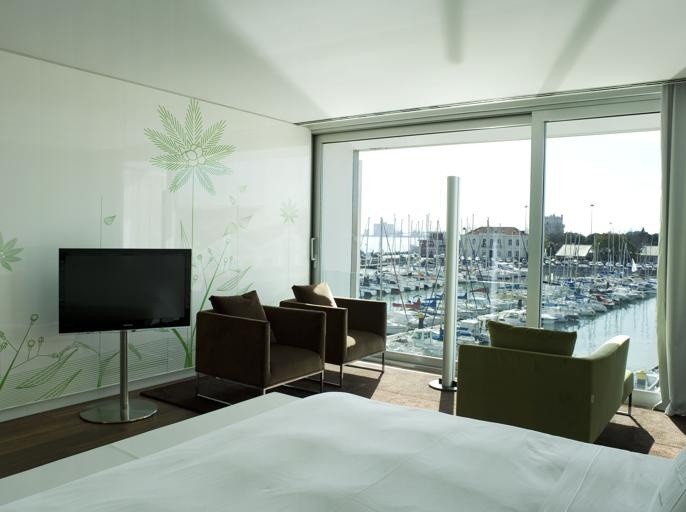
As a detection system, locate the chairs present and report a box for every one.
[196,305,327,405]
[280,297,386,388]
[457,335,633,444]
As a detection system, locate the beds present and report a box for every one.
[0,391,686,512]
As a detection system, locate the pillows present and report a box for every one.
[486,319,577,355]
[209,290,276,342]
[292,279,337,307]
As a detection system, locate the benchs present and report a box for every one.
[0,392,302,505]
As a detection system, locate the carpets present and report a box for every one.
[140,370,378,414]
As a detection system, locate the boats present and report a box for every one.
[631,366,660,393]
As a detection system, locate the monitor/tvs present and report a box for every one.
[58,248,191,332]
